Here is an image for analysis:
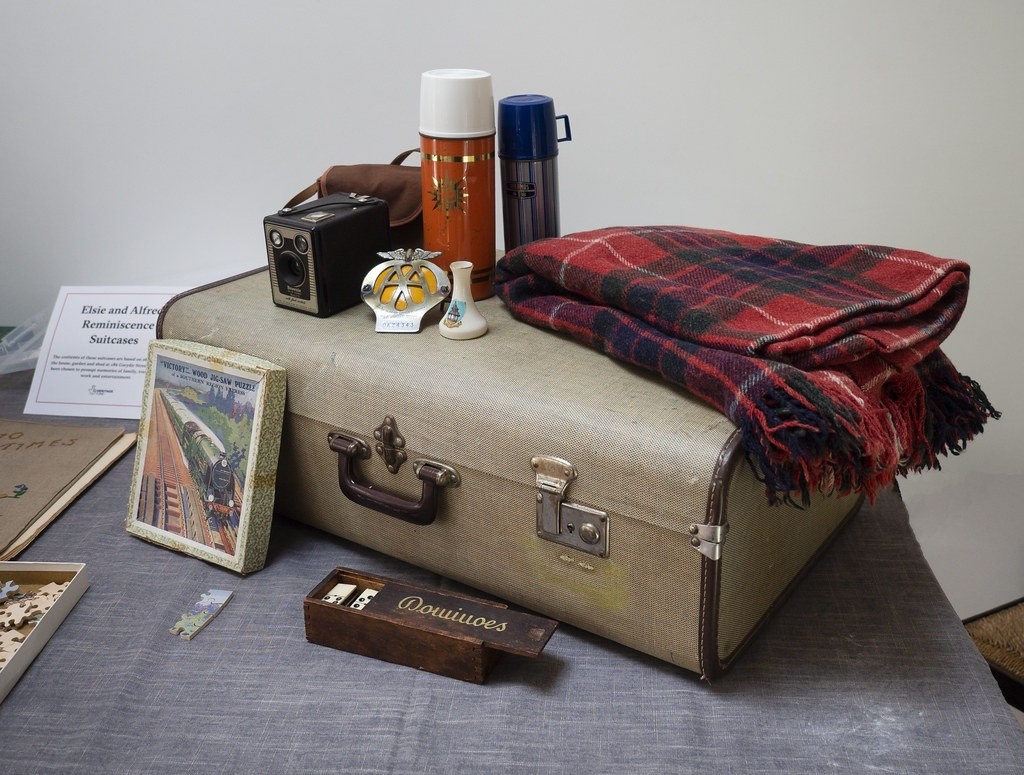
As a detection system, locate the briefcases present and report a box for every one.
[154,248,866,683]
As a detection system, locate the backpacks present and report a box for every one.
[316,145,436,253]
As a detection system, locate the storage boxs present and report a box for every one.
[280,69,573,304]
[157,251,867,687]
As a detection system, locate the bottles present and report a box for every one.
[438,261,489,342]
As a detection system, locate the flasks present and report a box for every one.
[493,93,569,254]
[419,68,498,304]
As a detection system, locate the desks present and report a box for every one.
[1,326,1024,775]
